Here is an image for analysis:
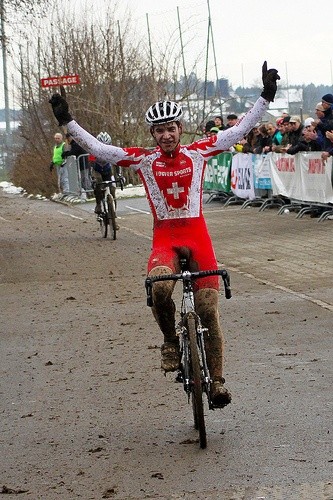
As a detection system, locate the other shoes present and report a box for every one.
[209,378,232,408]
[96,204,101,214]
[160,342,180,371]
[112,221,120,230]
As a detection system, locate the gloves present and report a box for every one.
[288,144,300,154]
[261,61,280,102]
[49,85,73,126]
[61,152,66,159]
[119,176,126,184]
[90,179,97,189]
[50,162,54,172]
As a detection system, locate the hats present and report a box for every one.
[276,118,283,125]
[252,123,260,129]
[316,102,326,111]
[227,115,237,119]
[210,127,219,132]
[283,116,290,122]
[304,118,314,126]
[321,94,333,104]
[289,116,300,122]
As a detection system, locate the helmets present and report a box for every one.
[144,101,183,126]
[97,131,112,145]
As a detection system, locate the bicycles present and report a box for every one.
[144,259,232,450]
[96,179,123,239]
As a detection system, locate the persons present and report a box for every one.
[50,61,280,409]
[62,133,92,197]
[205,94,333,219]
[89,132,120,230]
[49,132,69,193]
